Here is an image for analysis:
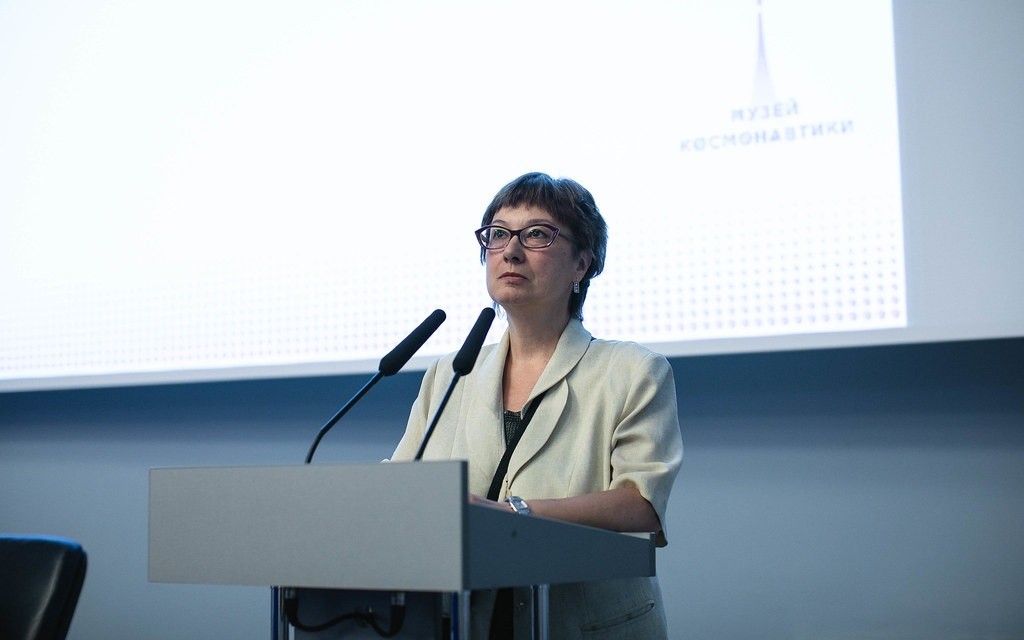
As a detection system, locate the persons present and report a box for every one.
[385,172,684,640]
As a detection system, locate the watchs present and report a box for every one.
[505,496,532,516]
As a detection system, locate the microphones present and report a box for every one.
[415,307,496,461]
[305,310,447,464]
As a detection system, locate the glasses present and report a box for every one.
[475,223,575,250]
